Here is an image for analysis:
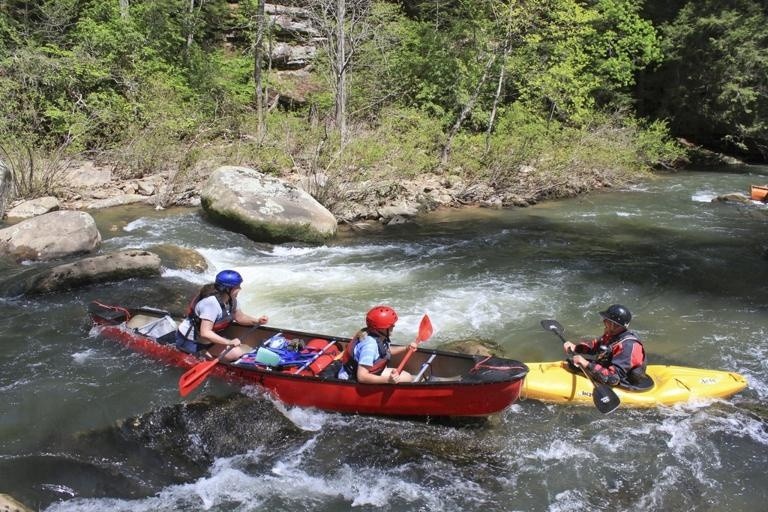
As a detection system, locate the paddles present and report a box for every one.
[178,318,268,397]
[542,318,620,415]
[394,314,433,376]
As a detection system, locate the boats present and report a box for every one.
[86,299,533,416]
[519,359,749,411]
[751,183,768,203]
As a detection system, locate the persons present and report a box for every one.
[563,304,649,387]
[337,305,463,384]
[175,269,270,364]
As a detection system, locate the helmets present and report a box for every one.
[366,306,398,329]
[598,304,632,330]
[216,270,243,288]
